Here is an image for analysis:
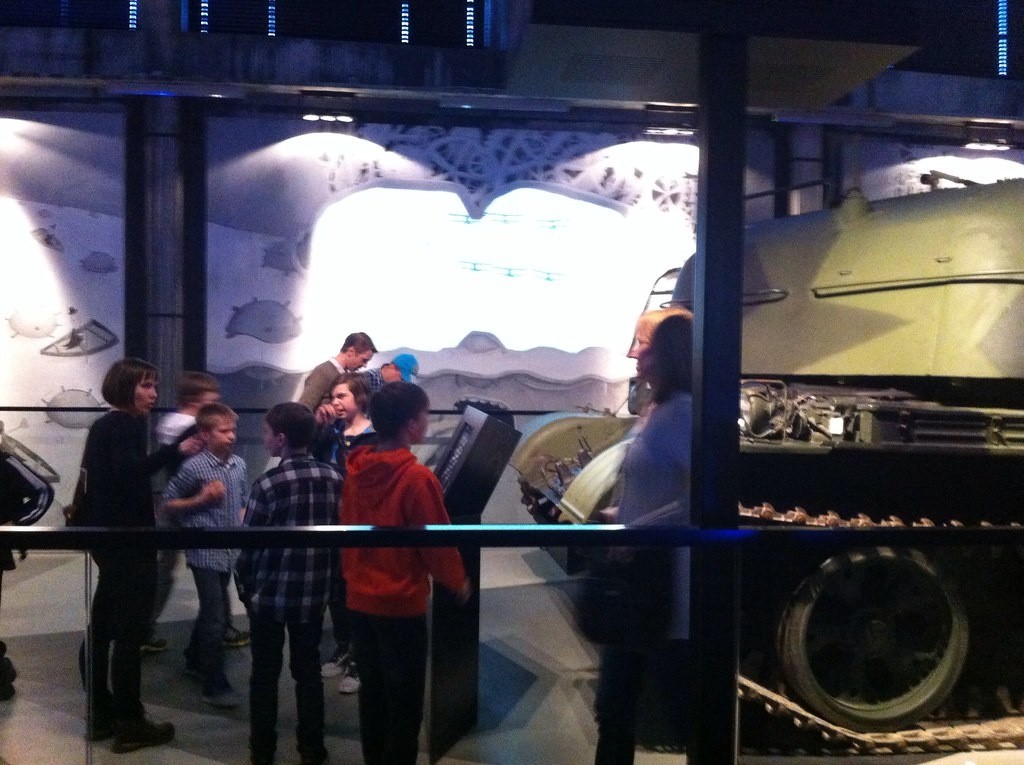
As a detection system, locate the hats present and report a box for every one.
[391,353,419,385]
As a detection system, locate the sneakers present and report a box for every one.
[339,661,360,693]
[219,626,251,646]
[140,635,168,651]
[322,645,354,677]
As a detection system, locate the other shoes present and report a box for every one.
[184,658,203,677]
[202,687,242,705]
[112,718,173,753]
[84,711,113,740]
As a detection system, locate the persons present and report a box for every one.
[340,382,472,765]
[592,305,692,765]
[298,332,418,429]
[67,358,179,753]
[314,374,378,694]
[135,372,250,654]
[0,422,54,702]
[231,403,344,765]
[159,404,249,708]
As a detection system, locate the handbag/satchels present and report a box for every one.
[574,552,677,645]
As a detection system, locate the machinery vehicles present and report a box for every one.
[507,163,1024,756]
